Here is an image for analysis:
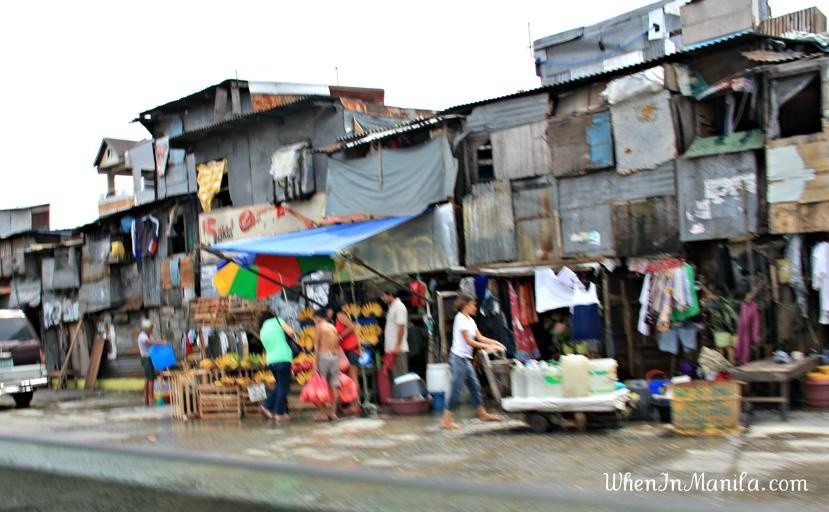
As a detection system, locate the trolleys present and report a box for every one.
[477,347,630,434]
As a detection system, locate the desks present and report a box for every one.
[731,356,819,420]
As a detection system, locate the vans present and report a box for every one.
[0,307,48,407]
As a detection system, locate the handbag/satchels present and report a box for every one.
[286,333,302,357]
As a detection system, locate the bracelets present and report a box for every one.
[338,334,343,341]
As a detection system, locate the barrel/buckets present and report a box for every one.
[149,343,177,370]
[426,362,453,395]
[509,350,618,397]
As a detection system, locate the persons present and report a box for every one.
[313,308,341,422]
[138,319,169,407]
[325,302,363,415]
[256,310,296,420]
[438,289,507,429]
[377,282,410,377]
